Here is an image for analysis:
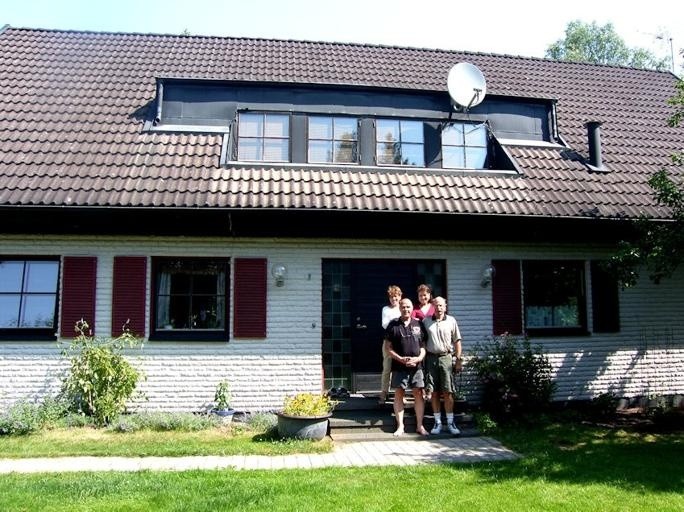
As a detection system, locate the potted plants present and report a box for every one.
[208,383,234,422]
[429,374,468,414]
[275,391,333,442]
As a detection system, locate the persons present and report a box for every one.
[411,284,436,402]
[377,285,409,405]
[384,298,430,436]
[421,296,463,435]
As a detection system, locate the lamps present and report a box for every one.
[272,264,287,287]
[480,264,496,288]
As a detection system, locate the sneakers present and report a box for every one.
[430,419,443,434]
[447,421,461,435]
